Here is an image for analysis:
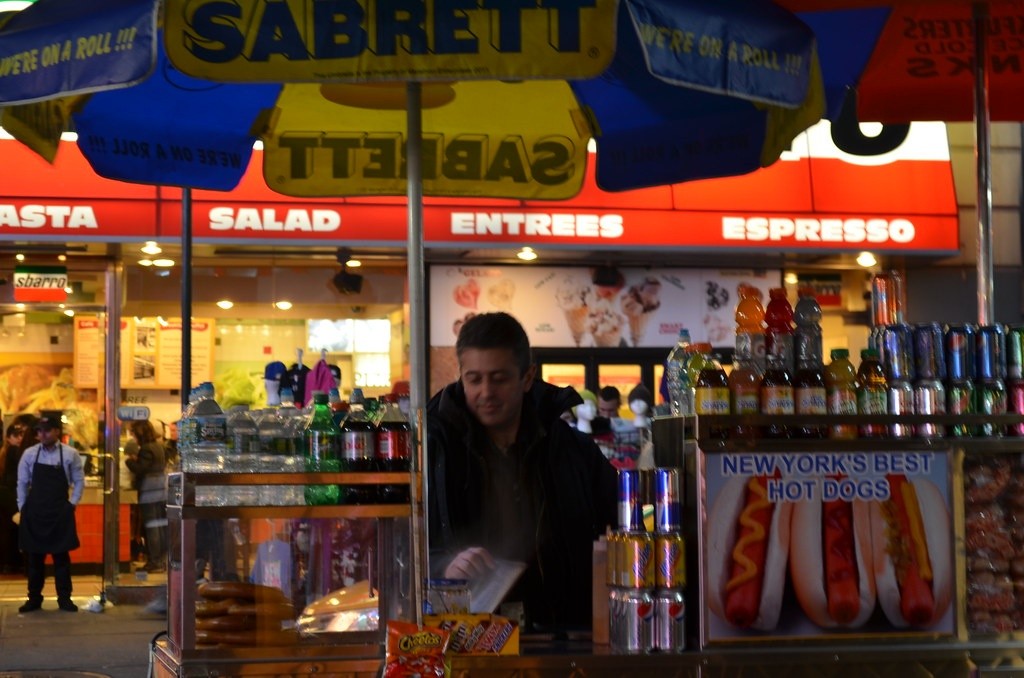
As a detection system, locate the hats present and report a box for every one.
[278,369,306,402]
[629,384,651,406]
[571,388,596,418]
[260,362,286,380]
[33,415,61,432]
[327,365,341,379]
[7,424,24,432]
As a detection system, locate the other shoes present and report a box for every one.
[195,559,206,577]
[19,600,38,612]
[59,599,78,612]
[136,559,166,573]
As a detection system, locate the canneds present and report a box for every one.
[872,270,1024,438]
[421,577,471,615]
[607,467,687,654]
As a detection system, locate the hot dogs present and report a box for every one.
[705,466,957,630]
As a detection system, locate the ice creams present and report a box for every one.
[555,265,662,348]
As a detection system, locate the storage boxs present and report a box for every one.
[422,614,520,657]
[592,549,610,645]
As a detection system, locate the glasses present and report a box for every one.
[14,431,23,437]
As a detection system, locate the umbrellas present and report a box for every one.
[775,0,1024,325]
[0,0,825,630]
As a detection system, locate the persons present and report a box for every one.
[597,386,622,417]
[427,313,619,641]
[0,414,41,575]
[569,389,597,434]
[124,419,226,619]
[628,383,651,427]
[264,361,341,406]
[16,414,84,614]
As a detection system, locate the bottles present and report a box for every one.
[177,382,411,506]
[592,524,613,644]
[665,286,888,437]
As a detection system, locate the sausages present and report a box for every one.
[193,580,302,646]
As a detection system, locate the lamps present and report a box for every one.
[594,263,619,287]
[332,245,362,296]
